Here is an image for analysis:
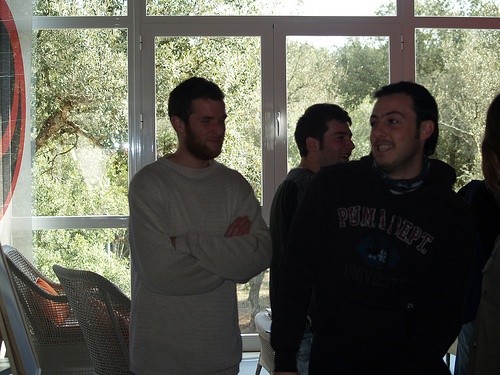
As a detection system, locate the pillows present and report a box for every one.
[33,277,70,325]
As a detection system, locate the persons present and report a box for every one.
[451,96,500,375]
[267,103,356,375]
[287,81,480,375]
[127,76,272,374]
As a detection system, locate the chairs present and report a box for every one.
[53,266,132,375]
[1,245,93,375]
[254,310,274,375]
